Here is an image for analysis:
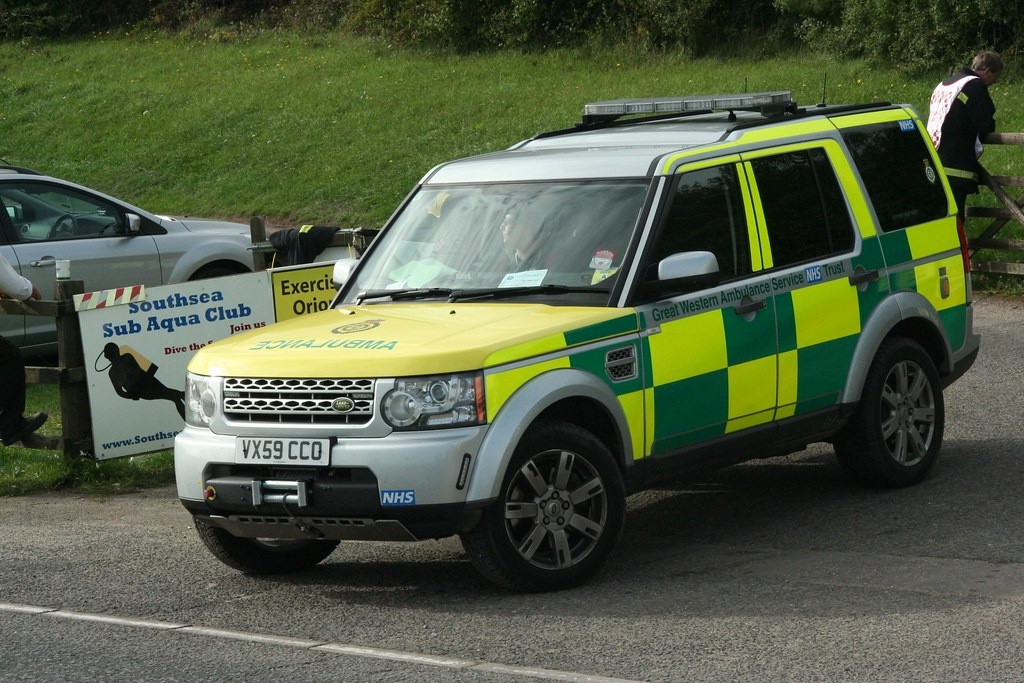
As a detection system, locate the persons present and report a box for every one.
[0,252,49,447]
[925,51,1003,227]
[476,202,562,275]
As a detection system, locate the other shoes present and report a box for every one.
[1,412,48,446]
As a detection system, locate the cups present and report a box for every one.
[55,260,71,281]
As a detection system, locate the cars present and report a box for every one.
[0,157,272,357]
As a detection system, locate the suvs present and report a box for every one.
[169,89,982,596]
